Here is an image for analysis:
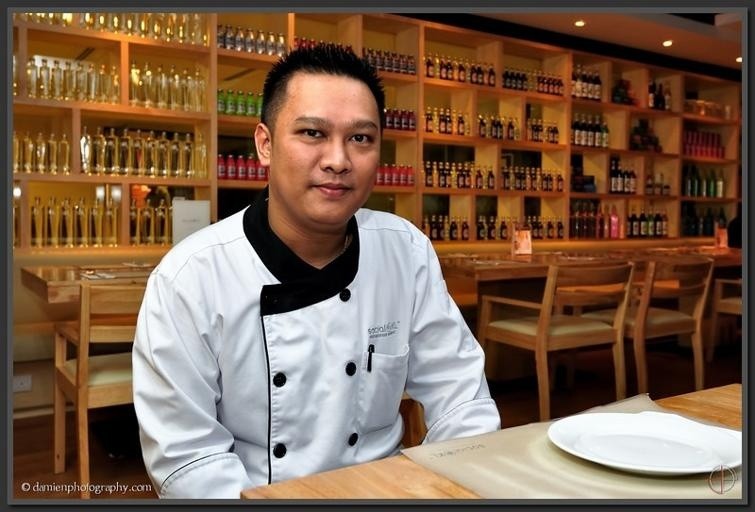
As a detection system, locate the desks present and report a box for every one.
[436,243,742,282]
[21,264,155,304]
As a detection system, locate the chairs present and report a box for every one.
[573,255,715,399]
[706,278,742,364]
[476,259,636,422]
[52,282,145,500]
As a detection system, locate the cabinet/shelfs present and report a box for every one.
[7,8,742,250]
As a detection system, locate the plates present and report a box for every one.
[547,409,743,480]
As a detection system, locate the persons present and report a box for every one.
[131,43,502,500]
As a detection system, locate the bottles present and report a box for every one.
[10,10,737,250]
